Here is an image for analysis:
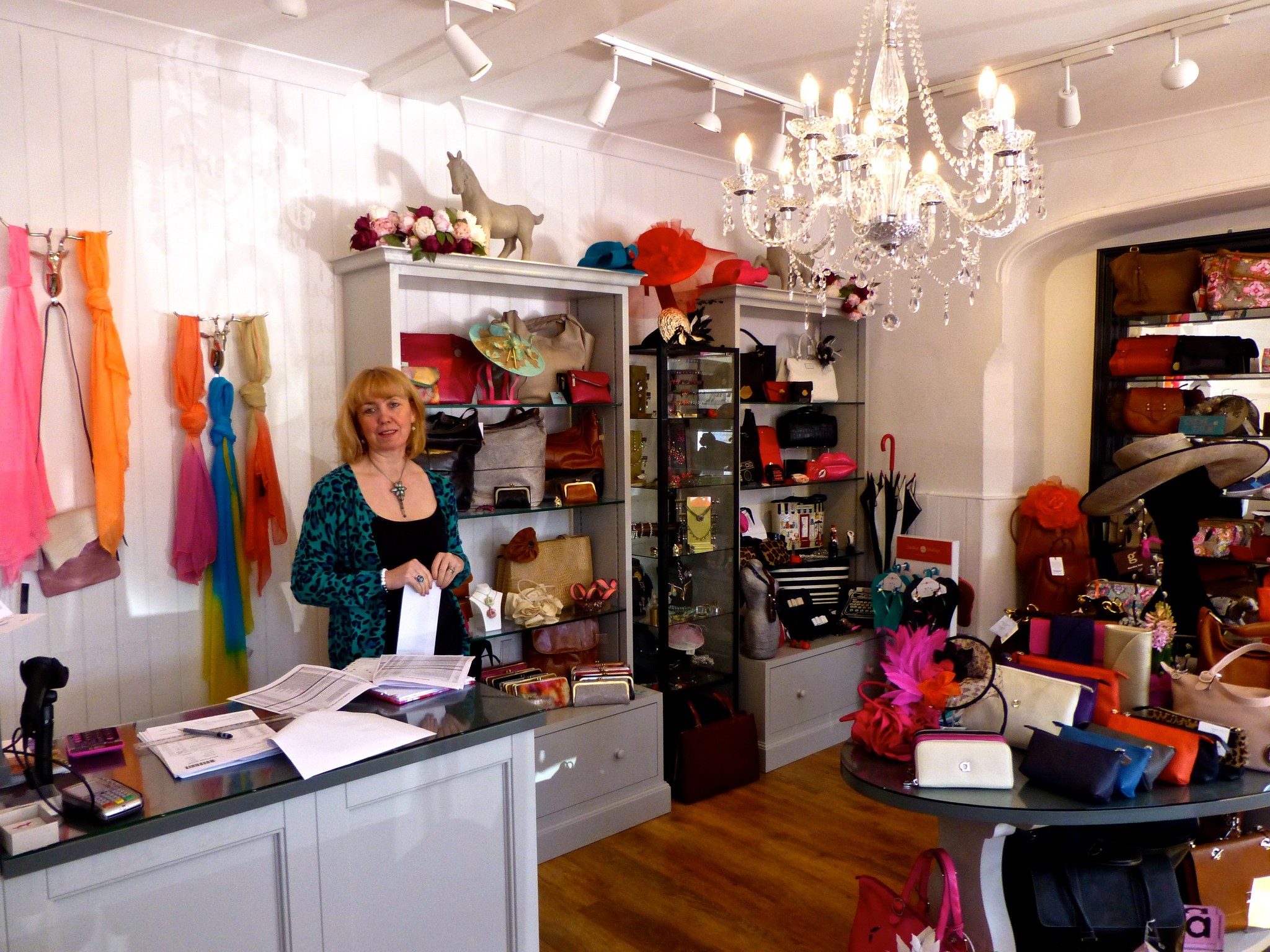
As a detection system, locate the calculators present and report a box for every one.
[65,727,127,757]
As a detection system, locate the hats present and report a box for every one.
[1078,430,1269,521]
[578,240,648,276]
[629,218,738,287]
[695,259,769,289]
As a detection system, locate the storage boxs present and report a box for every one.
[1,805,59,857]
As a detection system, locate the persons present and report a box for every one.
[740,558,780,659]
[290,366,472,670]
[417,685,476,739]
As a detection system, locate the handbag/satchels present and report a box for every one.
[840,587,875,627]
[846,847,972,952]
[1187,832,1270,931]
[1021,849,1185,951]
[30,503,121,598]
[527,618,600,676]
[398,308,594,404]
[739,506,790,570]
[407,408,605,507]
[740,327,839,403]
[1007,246,1270,774]
[738,406,839,484]
[494,534,594,618]
[672,692,759,805]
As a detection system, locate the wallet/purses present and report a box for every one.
[763,380,813,404]
[495,483,531,508]
[480,661,634,712]
[765,461,784,485]
[560,482,598,505]
[556,368,612,405]
[885,553,1247,806]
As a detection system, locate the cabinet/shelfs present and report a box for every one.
[1088,228,1270,492]
[629,345,739,796]
[327,245,675,865]
[697,284,886,773]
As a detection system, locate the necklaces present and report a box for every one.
[631,373,717,619]
[367,453,407,518]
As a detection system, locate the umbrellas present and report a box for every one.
[860,434,922,574]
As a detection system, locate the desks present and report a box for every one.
[0,680,548,952]
[840,735,1270,952]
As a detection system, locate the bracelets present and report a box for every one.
[381,568,388,593]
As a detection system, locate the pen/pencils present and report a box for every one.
[178,727,233,740]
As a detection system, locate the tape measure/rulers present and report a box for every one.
[134,712,294,751]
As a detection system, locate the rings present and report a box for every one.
[416,575,424,582]
[447,566,454,574]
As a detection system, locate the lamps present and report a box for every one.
[442,0,493,82]
[264,0,308,19]
[1161,37,1200,90]
[950,107,980,150]
[693,88,722,133]
[583,55,620,127]
[764,111,788,173]
[723,0,1047,331]
[1056,65,1082,128]
